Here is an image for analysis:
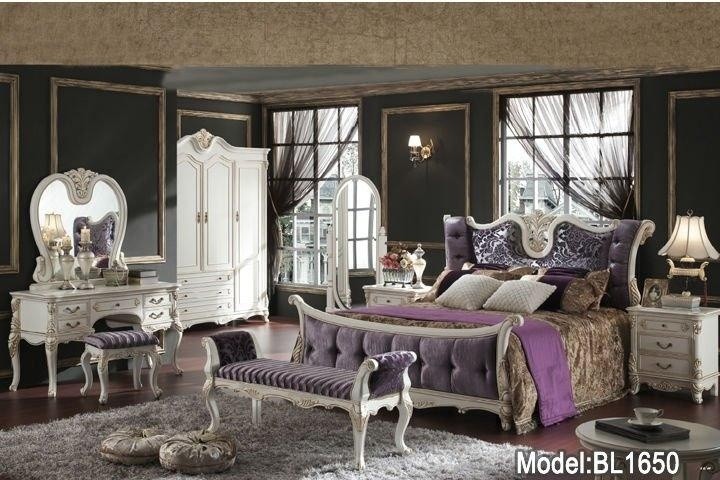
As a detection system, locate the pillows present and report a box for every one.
[421,262,611,315]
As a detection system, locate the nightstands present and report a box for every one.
[362,285,432,309]
[625,305,720,405]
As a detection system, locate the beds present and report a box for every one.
[288,210,655,434]
[74,212,119,258]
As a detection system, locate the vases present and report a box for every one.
[380,269,415,288]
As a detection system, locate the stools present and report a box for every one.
[80,331,163,404]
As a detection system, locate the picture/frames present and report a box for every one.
[641,278,669,308]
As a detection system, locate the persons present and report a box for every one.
[650,287,657,299]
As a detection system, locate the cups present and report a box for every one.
[633,407,664,424]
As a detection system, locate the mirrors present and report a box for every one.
[326,175,388,314]
[29,168,128,291]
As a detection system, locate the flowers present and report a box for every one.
[379,242,414,272]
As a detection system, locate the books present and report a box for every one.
[595,419,690,443]
[129,269,157,285]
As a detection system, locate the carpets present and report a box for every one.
[0,388,552,480]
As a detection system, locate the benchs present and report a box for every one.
[202,331,417,471]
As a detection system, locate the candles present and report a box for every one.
[81,224,90,241]
[62,235,71,245]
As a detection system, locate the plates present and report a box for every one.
[628,418,663,428]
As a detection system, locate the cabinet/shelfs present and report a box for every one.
[176,129,271,331]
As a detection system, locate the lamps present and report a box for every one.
[42,213,71,275]
[658,209,720,307]
[408,134,433,168]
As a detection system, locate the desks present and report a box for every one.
[8,281,183,398]
[575,415,720,480]
